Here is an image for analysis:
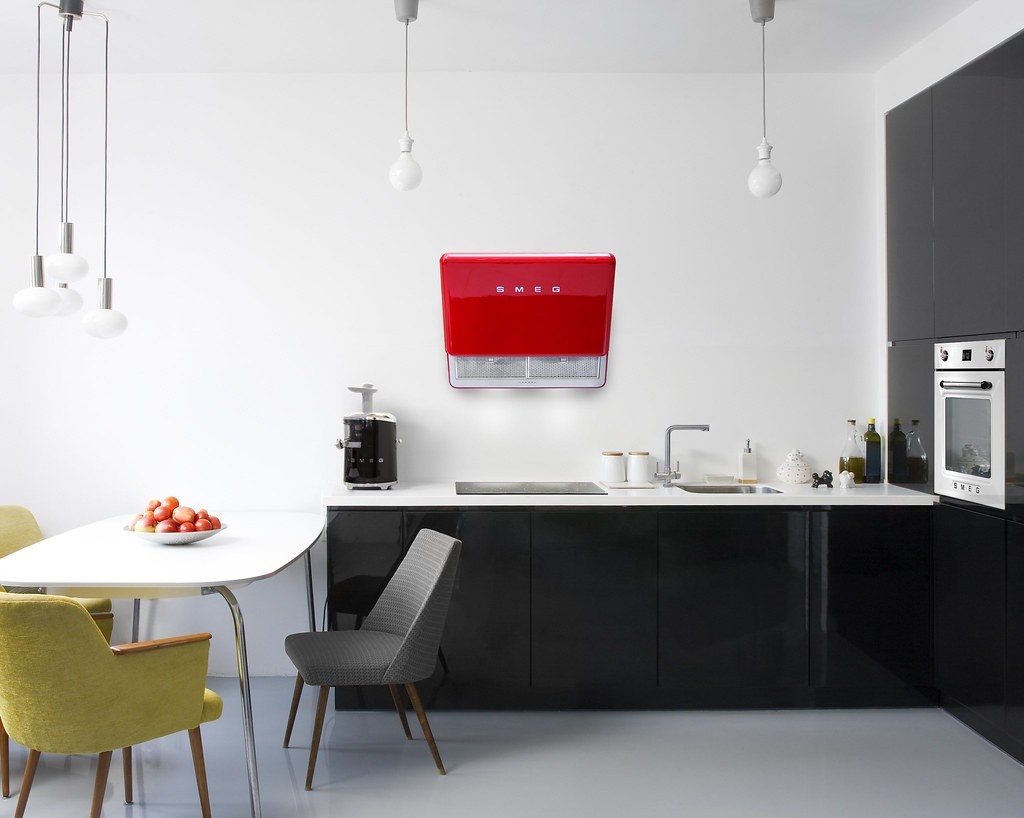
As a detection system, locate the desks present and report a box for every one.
[0,505,327,818]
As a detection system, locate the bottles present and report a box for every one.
[888,417,907,483]
[863,418,881,483]
[776,449,812,484]
[906,419,929,483]
[627,451,650,482]
[839,420,866,483]
[954,444,991,477]
[600,451,625,482]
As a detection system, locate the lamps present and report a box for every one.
[387,0,423,193]
[29,0,115,312]
[746,0,781,203]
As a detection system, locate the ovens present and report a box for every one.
[932,336,1005,511]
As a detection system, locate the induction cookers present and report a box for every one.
[454,481,609,494]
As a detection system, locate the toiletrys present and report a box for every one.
[738,438,758,484]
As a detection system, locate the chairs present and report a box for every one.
[0,503,112,799]
[0,592,222,818]
[283,528,462,790]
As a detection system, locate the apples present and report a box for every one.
[129,496,220,533]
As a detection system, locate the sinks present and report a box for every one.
[676,485,784,494]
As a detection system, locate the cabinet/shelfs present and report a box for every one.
[882,25,1023,503]
[326,505,1023,770]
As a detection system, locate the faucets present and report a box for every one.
[653,424,711,486]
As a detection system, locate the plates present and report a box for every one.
[124,523,226,544]
